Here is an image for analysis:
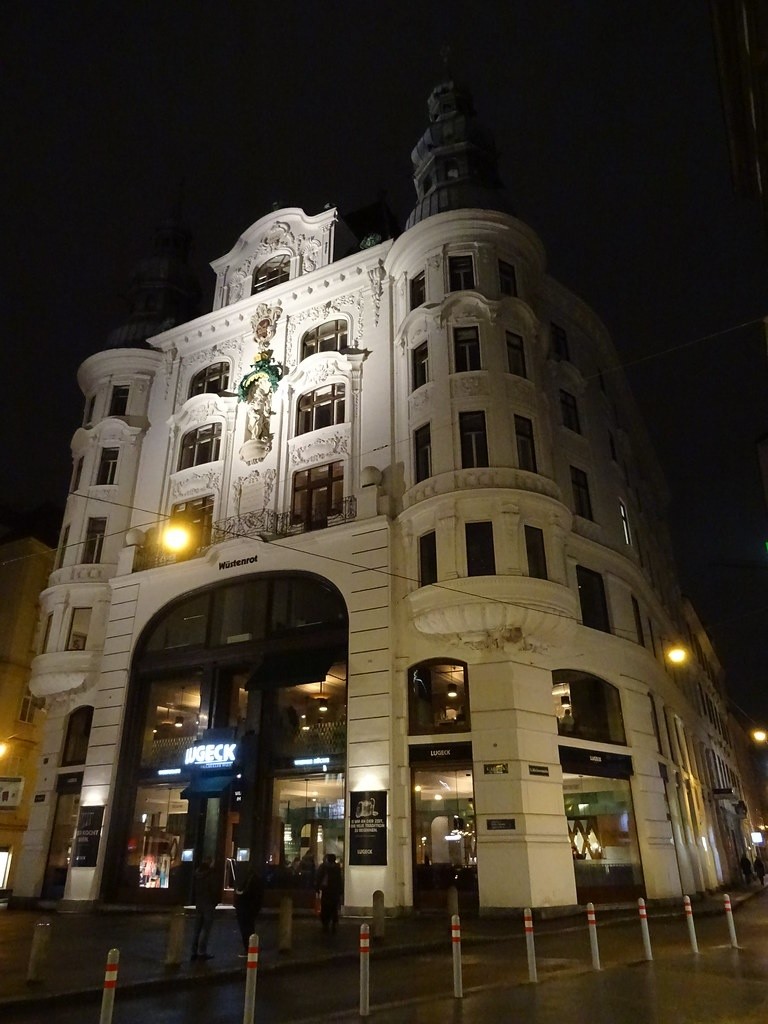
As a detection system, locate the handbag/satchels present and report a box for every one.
[317,864,329,889]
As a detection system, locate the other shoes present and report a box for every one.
[238,951,249,958]
[197,951,215,960]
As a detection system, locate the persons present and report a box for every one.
[314,853,343,933]
[753,856,765,886]
[308,717,327,754]
[741,854,752,884]
[170,745,180,765]
[190,870,222,960]
[233,863,264,958]
[561,709,574,733]
[148,858,168,888]
[248,389,271,442]
[330,714,347,753]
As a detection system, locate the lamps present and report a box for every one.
[318,682,327,711]
[454,771,464,830]
[175,686,185,727]
[448,665,457,698]
[560,685,570,708]
